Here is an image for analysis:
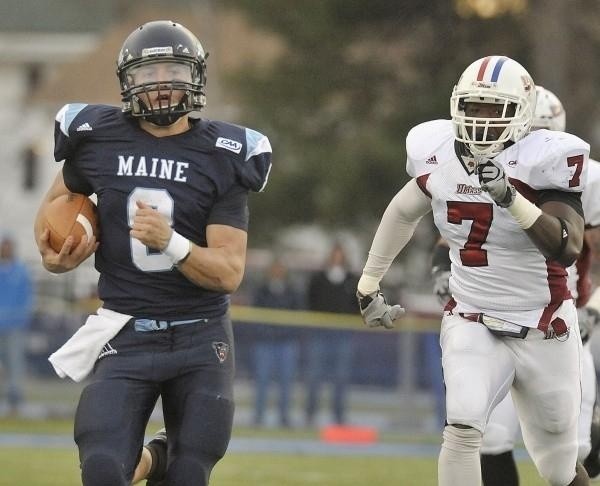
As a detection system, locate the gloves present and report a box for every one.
[433,271,450,305]
[474,156,515,208]
[356,288,406,329]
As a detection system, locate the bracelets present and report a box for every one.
[162,230,192,268]
[506,192,542,230]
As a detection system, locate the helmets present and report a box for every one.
[450,55,567,160]
[116,19,208,129]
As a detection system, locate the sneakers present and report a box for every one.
[145,428,166,486]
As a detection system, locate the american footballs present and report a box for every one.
[40,191,100,255]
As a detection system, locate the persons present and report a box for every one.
[480,88,600,485]
[33,20,274,486]
[349,53,591,485]
[250,234,297,430]
[301,238,361,425]
